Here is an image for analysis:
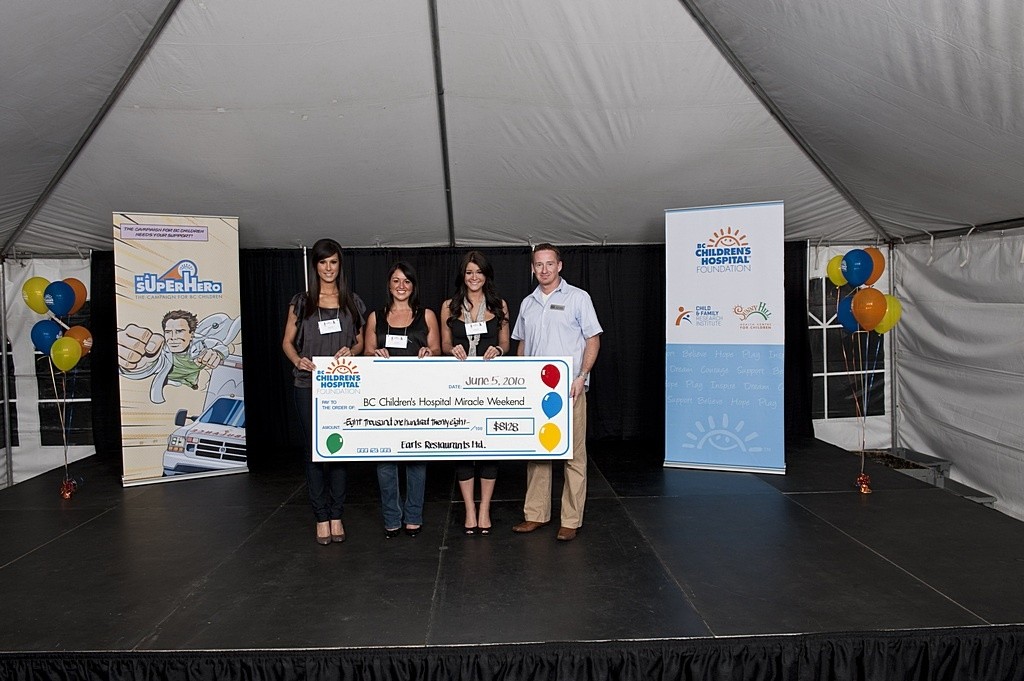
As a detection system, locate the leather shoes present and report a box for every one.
[512,519,544,532]
[317,536,331,545]
[557,525,576,540]
[331,534,346,542]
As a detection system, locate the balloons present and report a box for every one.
[826,248,901,336]
[21,276,93,373]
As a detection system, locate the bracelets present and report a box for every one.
[496,345,503,356]
[578,372,589,379]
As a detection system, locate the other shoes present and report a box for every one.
[385,527,400,539]
[406,526,420,537]
[479,526,493,536]
[465,525,478,536]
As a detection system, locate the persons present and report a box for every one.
[441,251,510,535]
[282,238,366,545]
[510,243,604,540]
[364,261,441,539]
